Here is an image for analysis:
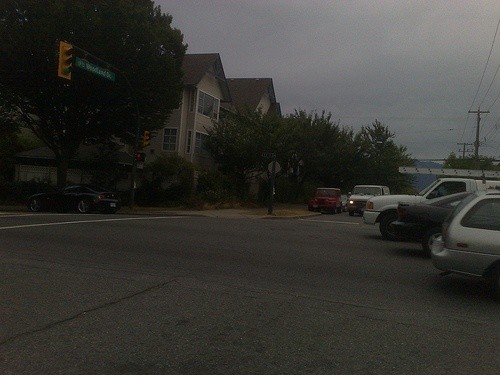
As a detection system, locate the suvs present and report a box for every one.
[348,185,390,216]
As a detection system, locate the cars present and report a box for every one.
[390,185,500,299]
[308,187,348,214]
[27,183,120,214]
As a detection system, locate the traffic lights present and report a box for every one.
[143,130,151,146]
[57,41,74,81]
[136,152,146,162]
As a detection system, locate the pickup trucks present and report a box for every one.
[363,178,500,241]
